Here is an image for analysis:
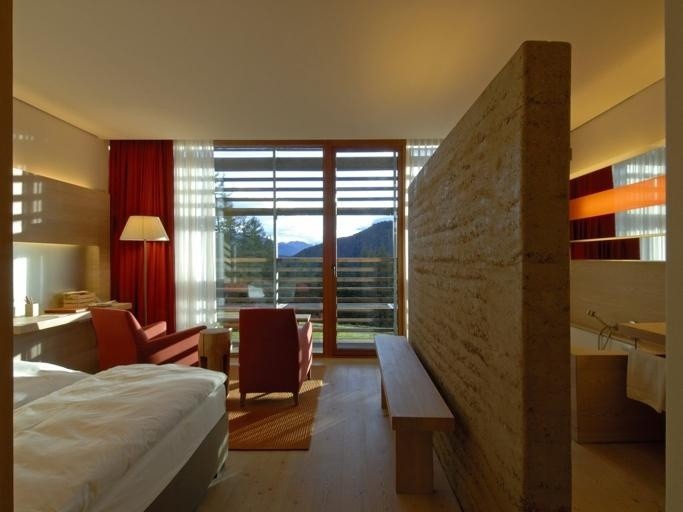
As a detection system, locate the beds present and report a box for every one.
[13,359,229,512]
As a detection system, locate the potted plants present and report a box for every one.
[24,296,39,316]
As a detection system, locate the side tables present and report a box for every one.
[198,327,231,398]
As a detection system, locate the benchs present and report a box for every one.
[373,333,456,495]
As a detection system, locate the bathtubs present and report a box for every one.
[570,325,635,445]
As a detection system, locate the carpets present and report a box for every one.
[226,364,327,451]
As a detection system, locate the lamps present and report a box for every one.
[119,215,170,327]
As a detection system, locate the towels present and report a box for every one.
[626,350,665,414]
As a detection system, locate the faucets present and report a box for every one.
[586,309,616,334]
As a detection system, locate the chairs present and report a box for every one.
[89,307,206,372]
[239,308,313,407]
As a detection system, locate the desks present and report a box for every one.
[618,322,666,347]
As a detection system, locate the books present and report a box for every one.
[44,290,132,313]
